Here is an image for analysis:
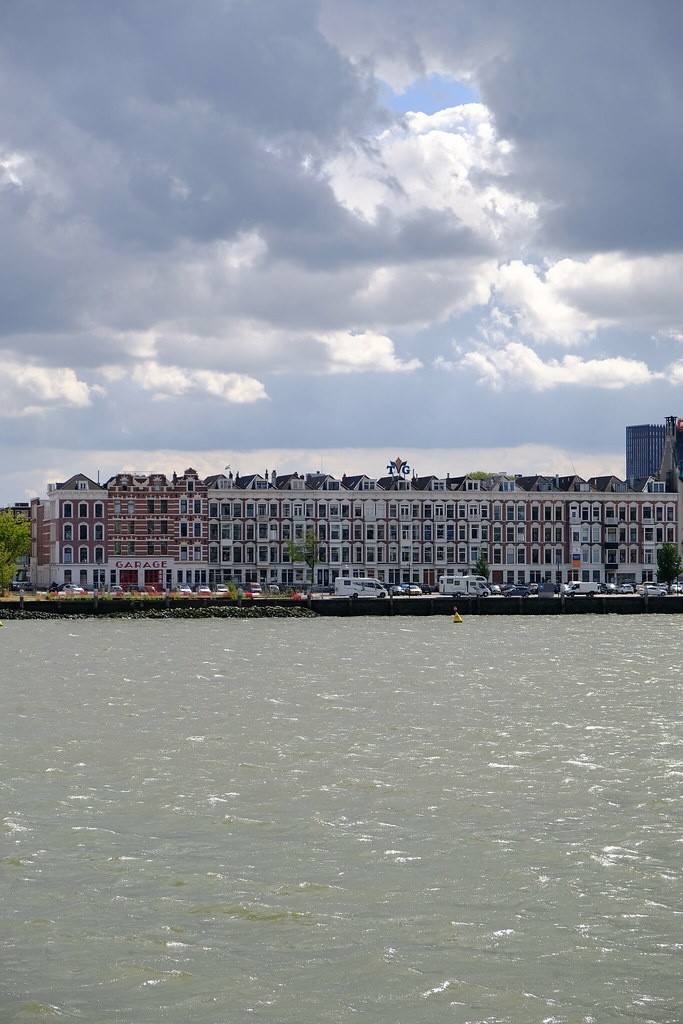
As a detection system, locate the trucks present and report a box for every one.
[334,576,388,598]
[438,575,491,598]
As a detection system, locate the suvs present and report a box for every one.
[500,586,531,597]
[564,581,599,598]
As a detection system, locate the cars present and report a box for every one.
[175,585,193,594]
[381,582,431,596]
[49,582,94,593]
[600,583,619,594]
[490,582,539,595]
[110,586,123,592]
[125,584,140,593]
[8,580,33,592]
[618,584,634,594]
[639,586,667,596]
[196,585,212,594]
[636,581,683,593]
[246,582,262,593]
[267,584,279,594]
[215,584,229,593]
[142,586,155,593]
